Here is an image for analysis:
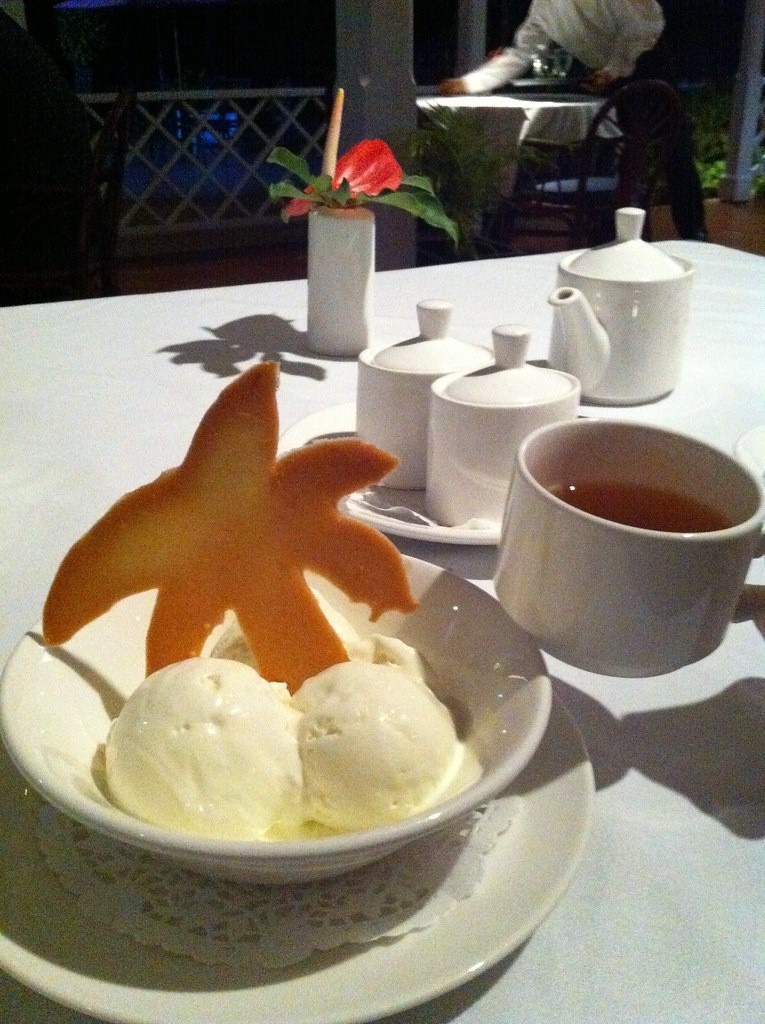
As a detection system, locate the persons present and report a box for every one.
[441,0,710,244]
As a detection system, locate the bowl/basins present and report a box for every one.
[3,550,554,884]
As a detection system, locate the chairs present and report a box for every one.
[490,78,679,255]
[0,85,142,309]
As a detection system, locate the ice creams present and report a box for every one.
[40,356,482,838]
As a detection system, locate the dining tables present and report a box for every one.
[0,236,765,1024]
[413,93,625,205]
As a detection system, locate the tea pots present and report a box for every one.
[547,207,697,406]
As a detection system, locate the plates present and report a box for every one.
[304,430,500,545]
[0,687,595,1022]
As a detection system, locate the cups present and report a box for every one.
[423,324,579,528]
[356,300,497,486]
[494,418,765,677]
[529,44,572,79]
